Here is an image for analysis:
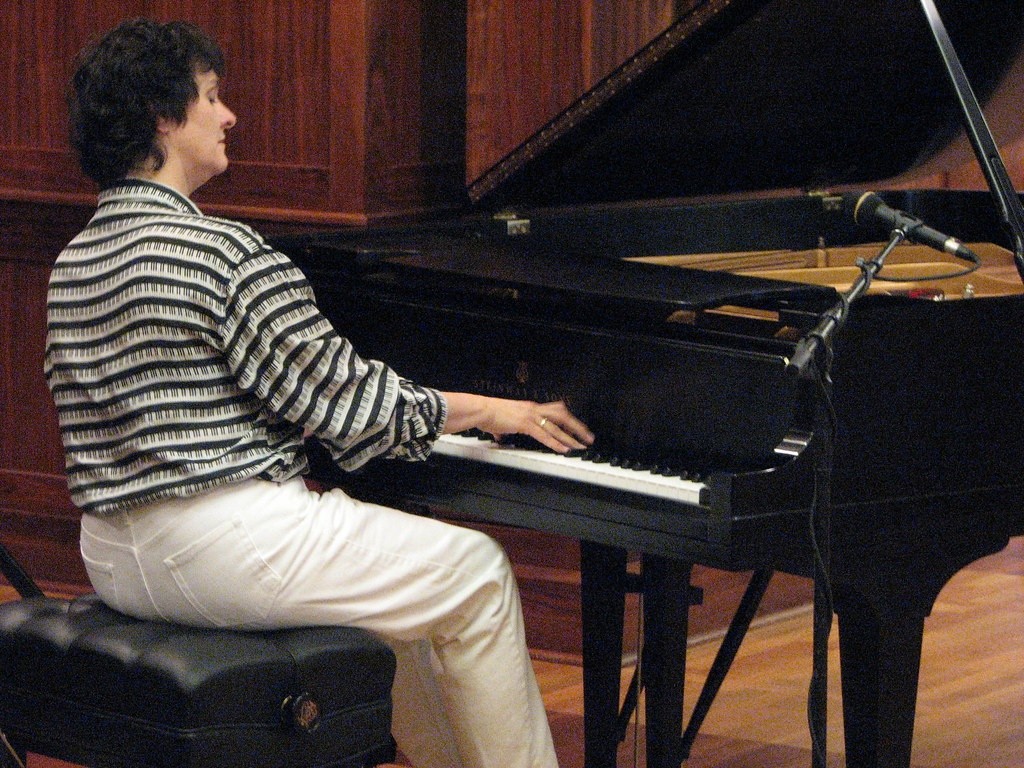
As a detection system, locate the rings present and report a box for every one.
[537,417,547,428]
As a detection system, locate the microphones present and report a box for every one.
[839,191,981,266]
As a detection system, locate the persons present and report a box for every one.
[44,16,593,768]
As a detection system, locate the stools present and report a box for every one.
[0,594,397,768]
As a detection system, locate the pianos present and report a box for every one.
[264,1,1024,768]
